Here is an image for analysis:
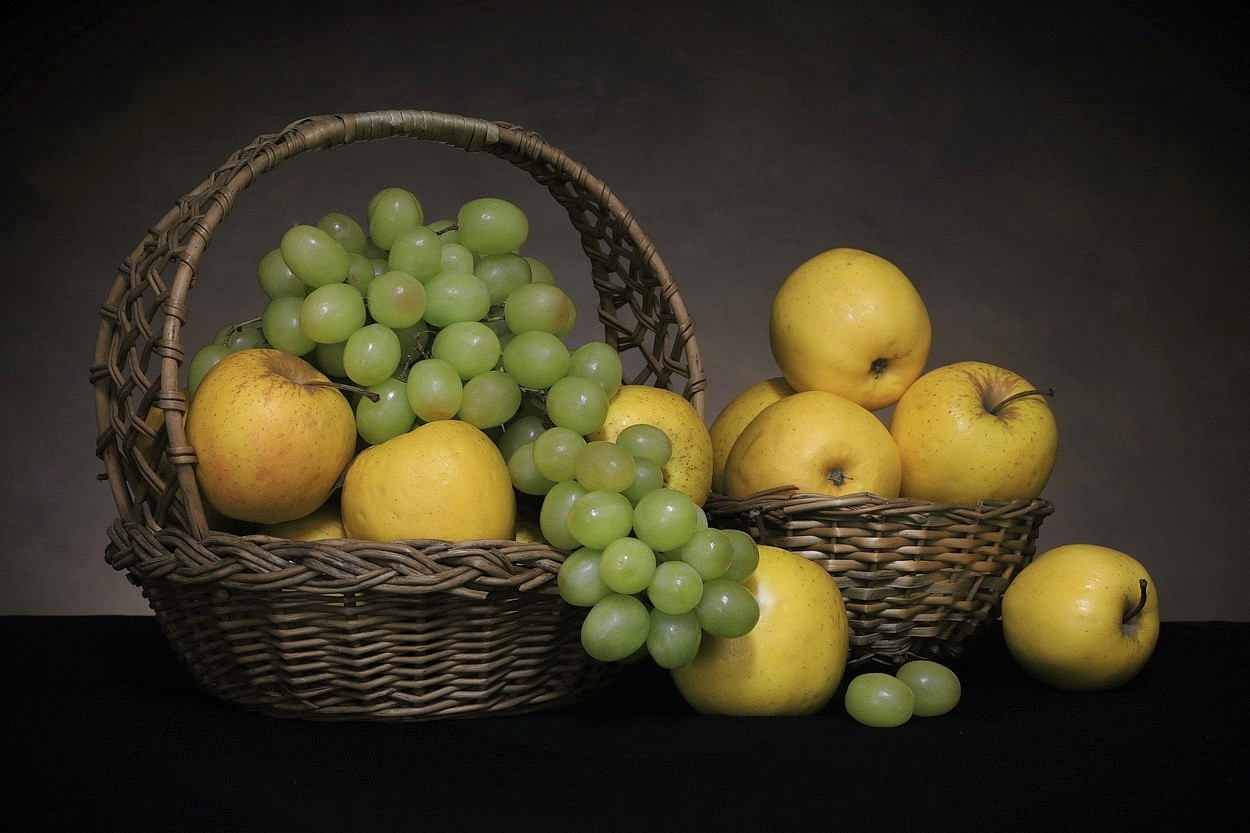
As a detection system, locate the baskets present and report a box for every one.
[706,490,1056,666]
[93,110,703,722]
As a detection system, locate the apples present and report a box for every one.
[710,248,1058,504]
[1000,545,1160,693]
[126,350,548,543]
[586,386,714,513]
[669,545,849,717]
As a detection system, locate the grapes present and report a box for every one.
[845,660,961,727]
[186,186,762,668]
